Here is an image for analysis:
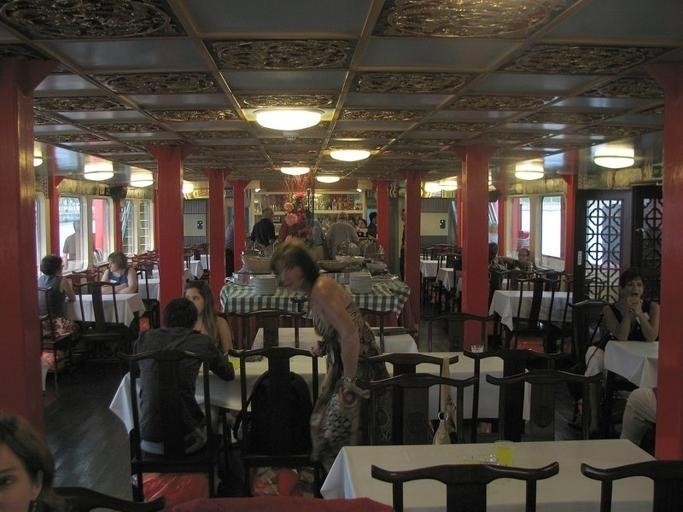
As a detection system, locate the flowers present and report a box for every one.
[278,173,314,244]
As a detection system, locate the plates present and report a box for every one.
[255,274,280,295]
[348,271,374,293]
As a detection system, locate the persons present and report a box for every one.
[583,267,659,438]
[183,281,233,352]
[489,241,522,276]
[516,245,534,279]
[271,240,393,482]
[62,220,96,271]
[133,298,234,455]
[619,386,658,445]
[37,254,81,338]
[100,253,138,294]
[0,413,75,512]
[225,206,406,282]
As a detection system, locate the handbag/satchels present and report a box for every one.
[566,361,587,402]
[433,357,458,445]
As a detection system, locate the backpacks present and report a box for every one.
[234,370,313,467]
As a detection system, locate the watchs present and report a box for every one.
[342,376,356,385]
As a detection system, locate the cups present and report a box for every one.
[483,457,498,466]
[471,345,483,354]
[228,351,239,371]
[494,441,513,466]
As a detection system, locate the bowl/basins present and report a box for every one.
[320,260,347,270]
[233,273,251,285]
